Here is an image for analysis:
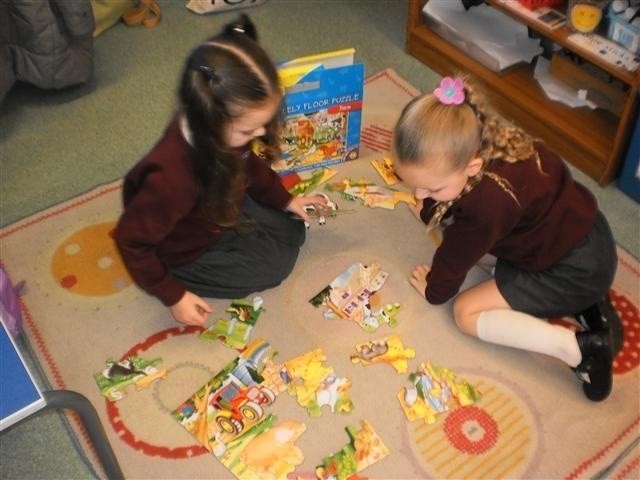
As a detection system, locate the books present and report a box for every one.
[497,0,567,32]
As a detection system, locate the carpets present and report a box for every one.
[0,71,640,480]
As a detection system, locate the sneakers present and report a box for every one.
[568,327,614,404]
[573,291,627,358]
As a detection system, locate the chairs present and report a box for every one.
[0,319,125,479]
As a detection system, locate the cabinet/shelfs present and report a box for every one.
[404,0,640,188]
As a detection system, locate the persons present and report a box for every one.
[385,69,626,403]
[113,11,332,326]
